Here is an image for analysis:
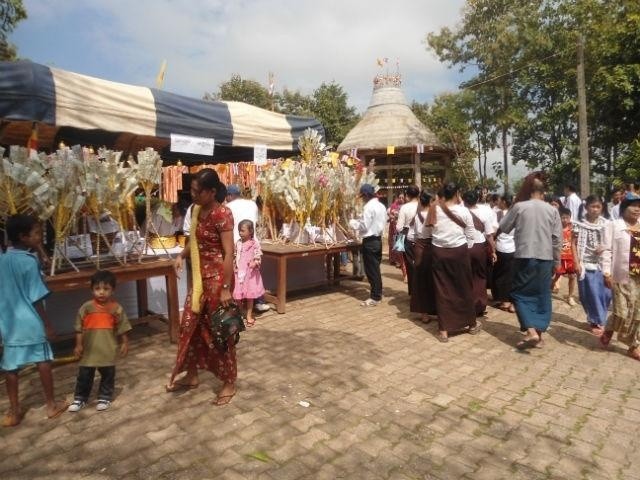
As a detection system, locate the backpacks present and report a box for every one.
[211,303,245,353]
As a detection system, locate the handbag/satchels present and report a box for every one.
[392,233,405,252]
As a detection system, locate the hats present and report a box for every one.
[226,185,241,195]
[359,184,374,194]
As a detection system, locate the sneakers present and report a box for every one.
[360,298,381,306]
[96,399,111,411]
[69,400,86,411]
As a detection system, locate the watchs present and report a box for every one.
[221,283,231,288]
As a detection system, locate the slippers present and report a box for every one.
[434,330,448,342]
[166,380,198,393]
[468,321,482,334]
[214,389,236,405]
[517,337,538,349]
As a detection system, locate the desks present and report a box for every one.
[44,254,182,344]
[259,238,362,314]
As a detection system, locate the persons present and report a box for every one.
[0,214,70,427]
[70,270,133,412]
[387,171,640,362]
[349,183,386,307]
[223,184,270,312]
[234,219,264,326]
[142,190,184,238]
[184,182,227,271]
[166,169,246,406]
